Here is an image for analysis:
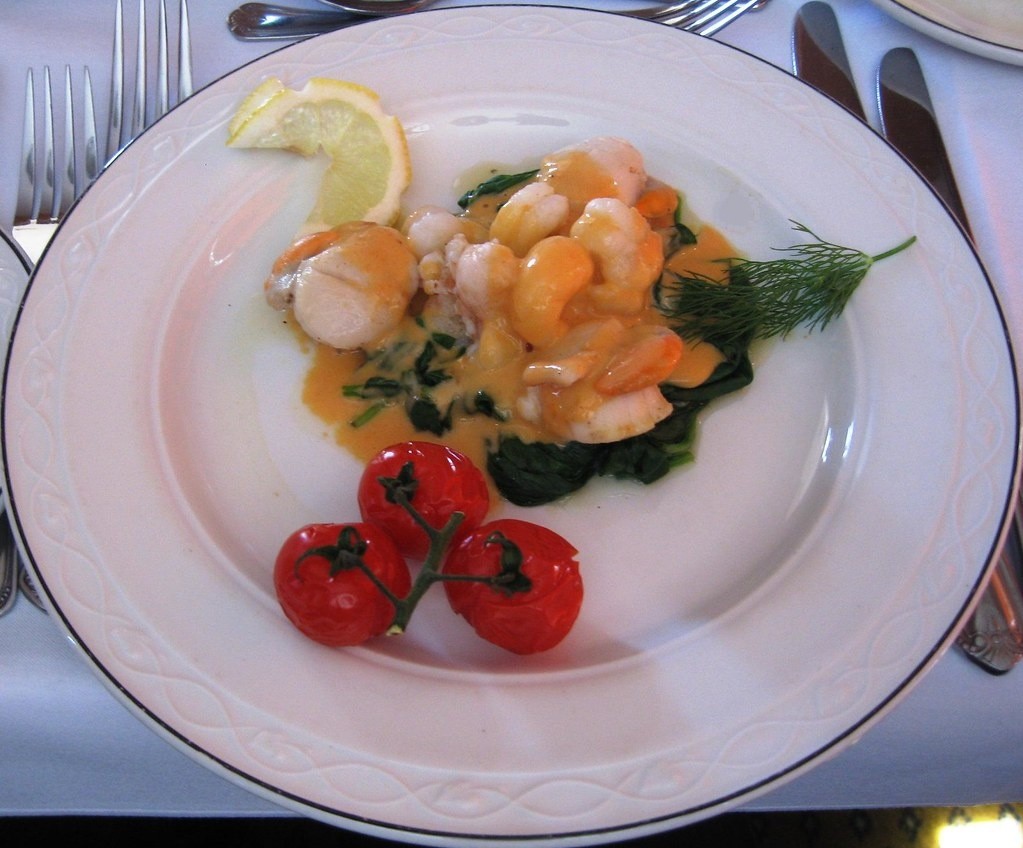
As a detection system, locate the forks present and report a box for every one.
[228,0,762,42]
[21,0,195,613]
[0,65,99,617]
[1,4,1021,848]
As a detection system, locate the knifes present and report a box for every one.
[876,47,976,248]
[790,1,1017,677]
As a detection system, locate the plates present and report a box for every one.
[878,0,1023,67]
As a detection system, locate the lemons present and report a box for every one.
[225,79,410,234]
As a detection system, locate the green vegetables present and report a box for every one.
[340,169,922,506]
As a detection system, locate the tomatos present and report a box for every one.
[272,443,585,654]
